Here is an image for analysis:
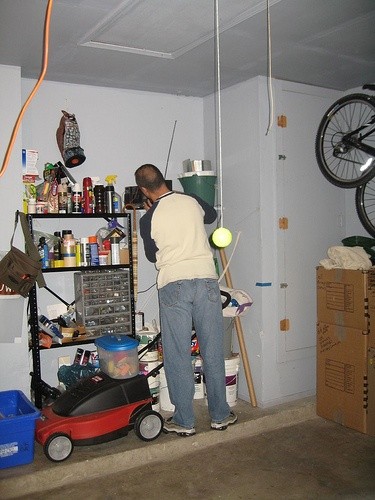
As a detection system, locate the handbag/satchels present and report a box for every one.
[0,211,46,298]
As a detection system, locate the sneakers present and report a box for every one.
[162,416,196,436]
[210,410,237,431]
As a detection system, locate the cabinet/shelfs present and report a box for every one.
[74,269,132,339]
[26,212,137,409]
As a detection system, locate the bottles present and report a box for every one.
[28,177,122,214]
[36,230,120,269]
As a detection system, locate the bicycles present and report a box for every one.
[314,80,375,242]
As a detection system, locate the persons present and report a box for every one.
[134,164,238,436]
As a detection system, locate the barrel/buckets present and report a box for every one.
[136,343,241,414]
[178,175,217,208]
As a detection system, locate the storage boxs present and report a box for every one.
[59,326,73,337]
[0,390,42,469]
[94,333,140,380]
[317,266,375,438]
